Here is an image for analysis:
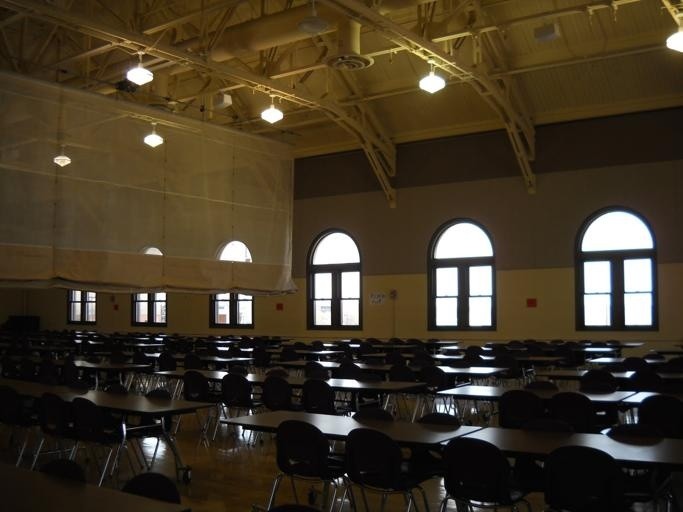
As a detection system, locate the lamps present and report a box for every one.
[666,13,683,54]
[143,122,164,148]
[126,50,154,86]
[261,95,283,123]
[419,64,445,94]
[54,145,71,167]
